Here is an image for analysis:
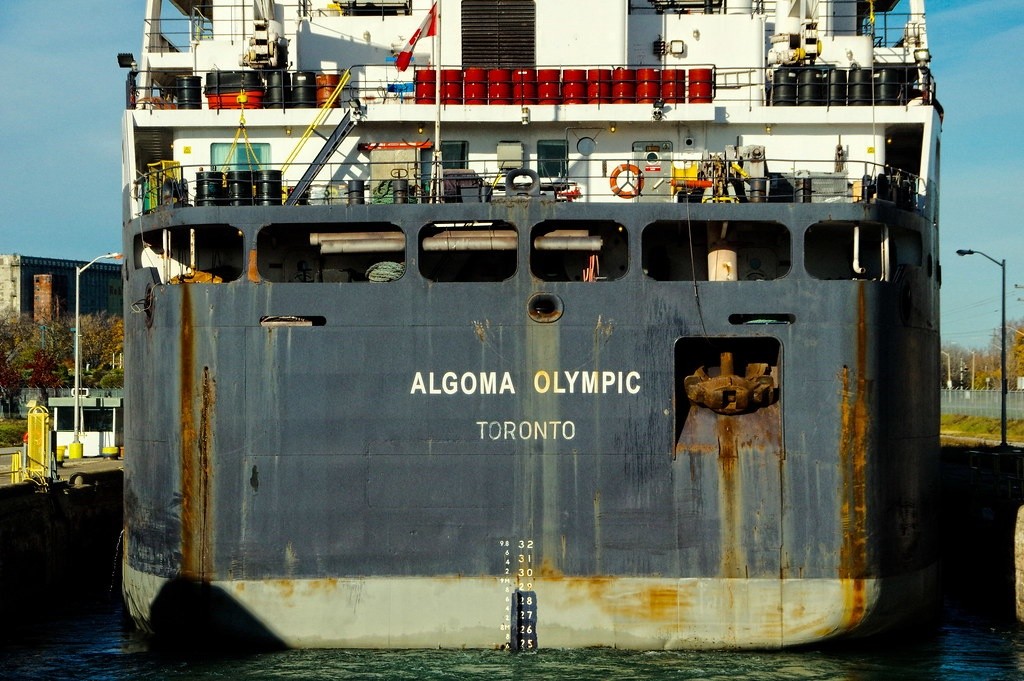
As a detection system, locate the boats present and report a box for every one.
[113,0,946,648]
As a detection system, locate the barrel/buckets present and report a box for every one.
[268,72,291,108]
[226,172,252,206]
[393,180,408,203]
[416,68,712,105]
[772,70,798,105]
[292,72,316,108]
[874,69,899,106]
[848,69,873,105]
[481,187,491,202]
[254,170,282,206]
[799,69,823,105]
[823,69,847,106]
[750,178,766,203]
[196,172,224,206]
[176,75,202,109]
[708,250,737,281]
[316,74,341,108]
[348,180,365,205]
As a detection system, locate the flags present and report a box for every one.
[394,2,437,72]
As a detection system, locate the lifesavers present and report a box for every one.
[610,164,646,199]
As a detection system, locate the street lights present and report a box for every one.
[75,252,122,441]
[952,342,976,391]
[957,248,1007,445]
